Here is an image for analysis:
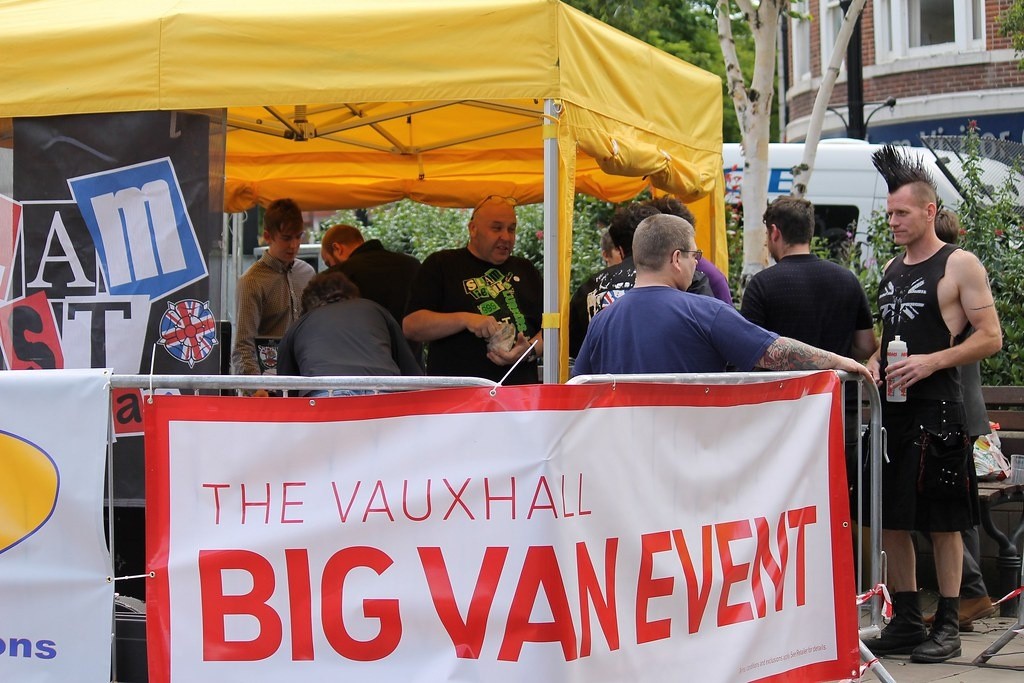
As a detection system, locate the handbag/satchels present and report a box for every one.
[971,419,1009,480]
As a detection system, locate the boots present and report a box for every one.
[911,594,963,663]
[859,594,924,655]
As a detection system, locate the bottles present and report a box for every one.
[887,334,907,404]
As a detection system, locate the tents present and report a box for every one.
[0,0,735,389]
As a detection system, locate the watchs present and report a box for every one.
[527,341,538,363]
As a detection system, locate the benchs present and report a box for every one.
[862,386,1024,618]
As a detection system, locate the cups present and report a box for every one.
[1010,454,1024,486]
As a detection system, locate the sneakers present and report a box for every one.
[921,589,996,631]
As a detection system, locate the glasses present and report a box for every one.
[669,244,704,264]
[466,194,519,217]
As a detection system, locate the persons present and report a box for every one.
[598,229,625,268]
[588,214,615,238]
[566,203,717,359]
[858,177,1004,664]
[737,193,878,453]
[321,219,425,367]
[273,268,428,396]
[916,207,1000,636]
[231,199,319,398]
[400,195,546,387]
[574,214,878,386]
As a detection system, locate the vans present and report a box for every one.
[721,138,1024,274]
